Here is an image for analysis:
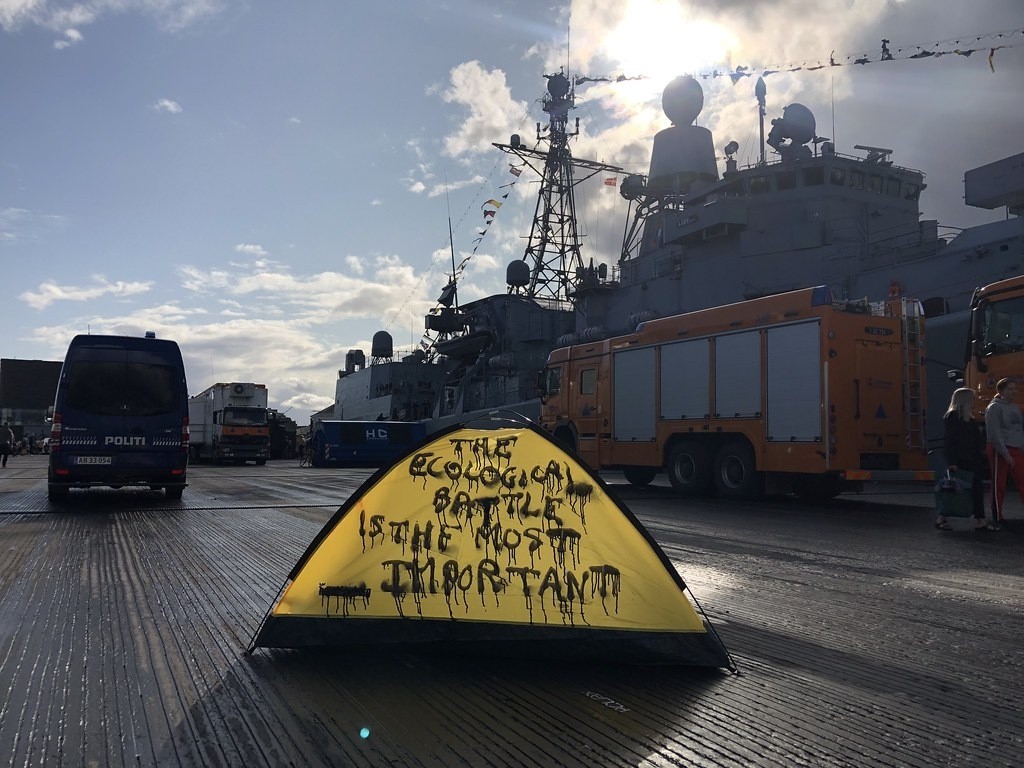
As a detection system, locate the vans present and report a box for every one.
[46,330,191,504]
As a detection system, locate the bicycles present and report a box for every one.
[299,445,318,468]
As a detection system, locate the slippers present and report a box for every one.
[934,520,954,531]
[974,522,1001,532]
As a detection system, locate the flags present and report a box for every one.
[605,177,616,186]
[420,164,525,350]
[576,39,994,86]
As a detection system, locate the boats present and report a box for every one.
[308,21,1024,449]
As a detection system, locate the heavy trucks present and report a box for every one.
[188,382,271,466]
[948,275,1024,488]
[537,283,938,510]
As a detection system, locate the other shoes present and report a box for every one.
[2,465,7,468]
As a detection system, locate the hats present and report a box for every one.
[288,440,292,443]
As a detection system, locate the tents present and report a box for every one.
[242,410,741,675]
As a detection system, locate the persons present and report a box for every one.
[985,378,1024,524]
[0,421,14,468]
[12,429,48,457]
[934,387,1000,532]
[298,435,305,460]
[283,436,293,459]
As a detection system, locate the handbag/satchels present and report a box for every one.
[944,462,978,497]
[942,469,956,491]
